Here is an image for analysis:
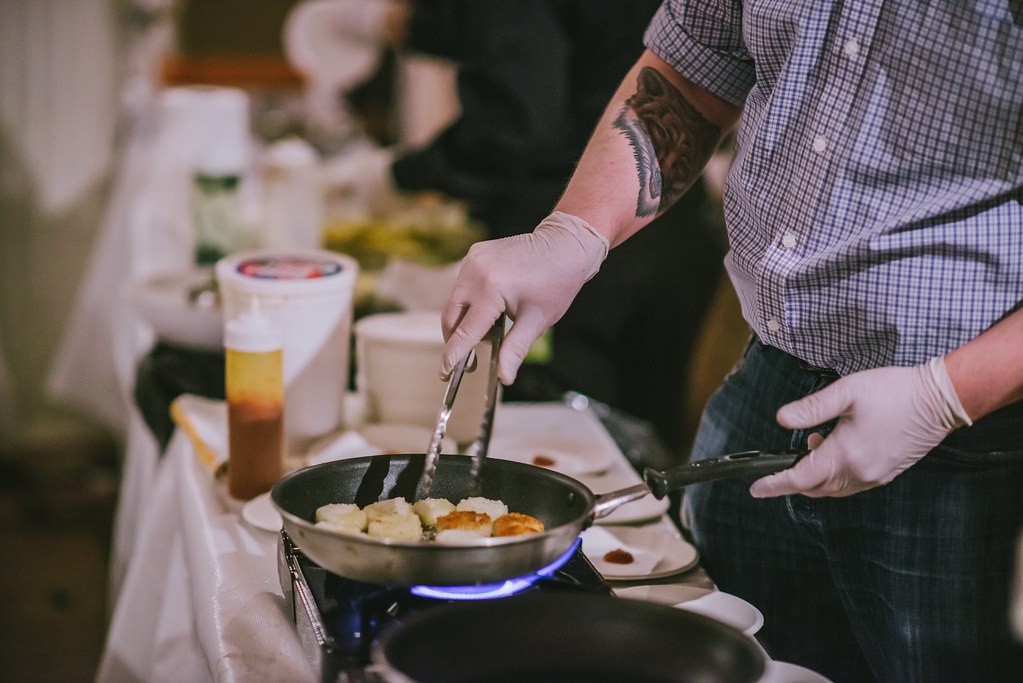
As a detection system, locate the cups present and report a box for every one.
[215,249,357,440]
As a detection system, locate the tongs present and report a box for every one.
[425,311,506,492]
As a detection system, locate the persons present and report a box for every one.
[437,0,1023,683]
[285,0,711,453]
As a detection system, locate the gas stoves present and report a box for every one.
[278,528,617,683]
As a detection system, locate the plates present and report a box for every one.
[755,661,835,683]
[306,424,458,467]
[578,526,700,580]
[242,490,283,533]
[468,433,612,476]
[580,476,672,525]
[612,585,764,637]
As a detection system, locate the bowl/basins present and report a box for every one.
[131,269,228,351]
[355,311,502,446]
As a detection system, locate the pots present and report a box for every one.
[372,590,771,683]
[270,449,813,588]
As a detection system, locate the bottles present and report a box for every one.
[224,300,284,500]
[262,135,324,253]
[195,171,239,265]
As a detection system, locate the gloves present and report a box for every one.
[441,210,609,383]
[750,358,974,499]
